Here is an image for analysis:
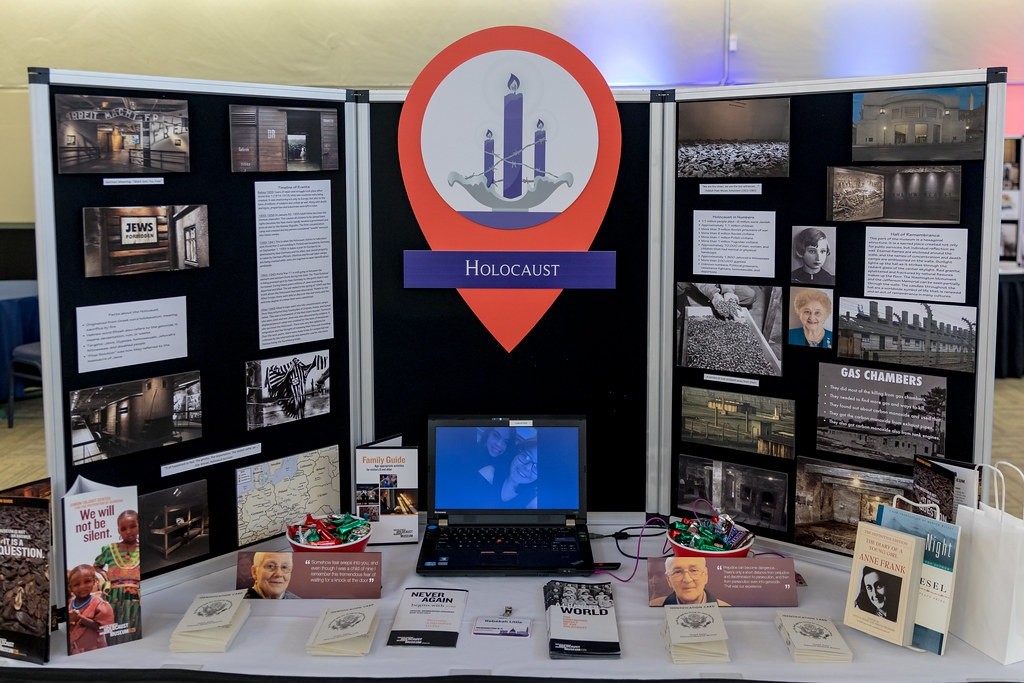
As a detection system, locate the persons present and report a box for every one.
[550,582,612,608]
[855,565,902,623]
[244,553,300,600]
[68,511,141,654]
[788,289,836,349]
[792,227,837,288]
[650,557,729,607]
[676,281,757,330]
[476,428,539,509]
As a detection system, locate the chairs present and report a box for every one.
[7,340,42,426]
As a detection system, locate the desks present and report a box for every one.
[0,280,41,419]
[0,525,1024,683]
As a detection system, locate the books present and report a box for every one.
[843,504,961,656]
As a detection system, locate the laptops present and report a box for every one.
[416,416,595,577]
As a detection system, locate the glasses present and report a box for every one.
[669,568,707,580]
[260,560,292,574]
